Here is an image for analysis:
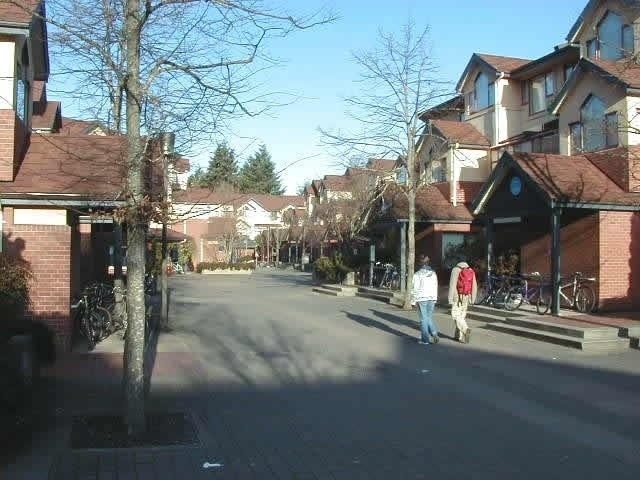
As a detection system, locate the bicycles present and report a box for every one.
[352,261,404,291]
[70,279,127,351]
[167,258,184,275]
[474,269,597,316]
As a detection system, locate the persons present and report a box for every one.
[410,253,440,345]
[447,254,478,344]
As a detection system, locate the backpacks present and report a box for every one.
[455,266,473,294]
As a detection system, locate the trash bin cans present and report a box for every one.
[7,334,42,391]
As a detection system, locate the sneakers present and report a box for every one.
[418,333,439,344]
[455,329,471,343]
